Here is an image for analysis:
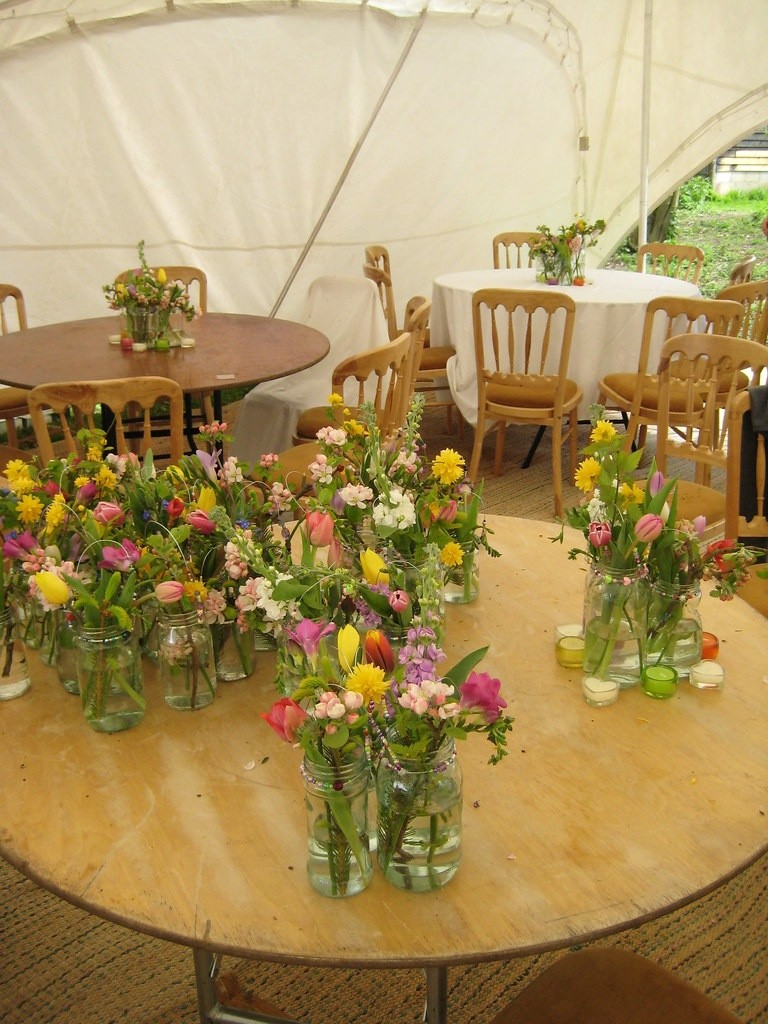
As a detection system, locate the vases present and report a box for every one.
[156,610,216,711]
[256,615,276,649]
[217,606,256,683]
[379,623,413,662]
[301,753,374,899]
[279,616,339,714]
[384,553,425,585]
[0,606,31,701]
[645,577,702,675]
[579,560,643,688]
[371,727,466,892]
[15,596,63,661]
[439,540,480,602]
[539,259,584,283]
[56,611,89,695]
[340,525,375,568]
[77,621,143,732]
[124,313,170,344]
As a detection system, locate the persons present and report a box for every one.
[739,218,768,566]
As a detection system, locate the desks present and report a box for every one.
[432,270,705,468]
[1,312,331,468]
[0,515,768,1024]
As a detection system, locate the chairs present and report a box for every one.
[494,231,542,271]
[366,242,465,437]
[466,289,582,514]
[28,377,183,473]
[213,945,744,1024]
[292,293,431,436]
[599,297,745,485]
[662,278,768,444]
[232,273,391,465]
[245,332,414,493]
[720,387,768,619]
[724,253,758,284]
[635,242,703,285]
[116,266,216,442]
[635,333,768,540]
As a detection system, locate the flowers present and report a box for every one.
[100,243,205,322]
[526,220,608,258]
[0,376,759,752]
